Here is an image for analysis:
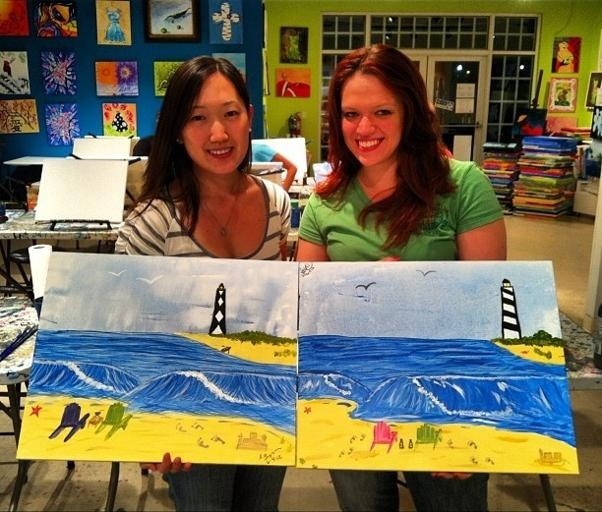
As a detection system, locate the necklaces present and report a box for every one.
[200,173,242,236]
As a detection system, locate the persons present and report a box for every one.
[292,40,507,507]
[112,52,290,508]
[555,89,570,106]
[285,113,312,176]
[104,3,127,42]
[557,41,574,73]
[250,143,298,192]
[278,72,297,98]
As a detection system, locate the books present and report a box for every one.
[479,132,576,218]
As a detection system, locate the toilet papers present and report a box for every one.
[27,244,53,300]
